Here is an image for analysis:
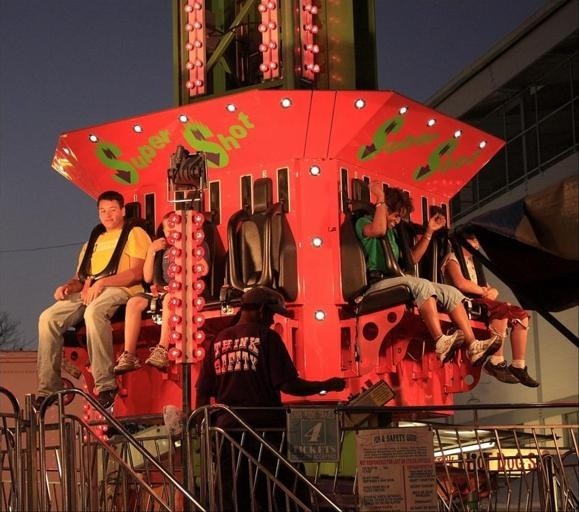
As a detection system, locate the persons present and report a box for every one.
[191,286,345,511]
[111,209,210,373]
[440,230,540,388]
[26,189,154,412]
[353,181,503,367]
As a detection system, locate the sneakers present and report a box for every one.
[468,335,503,368]
[114,352,142,373]
[98,387,120,411]
[145,345,170,369]
[435,328,465,363]
[507,362,540,387]
[485,358,520,384]
[35,391,55,412]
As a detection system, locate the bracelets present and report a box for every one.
[374,201,386,208]
[423,231,432,241]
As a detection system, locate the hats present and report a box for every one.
[241,287,294,319]
